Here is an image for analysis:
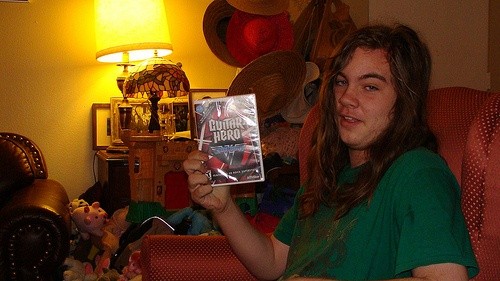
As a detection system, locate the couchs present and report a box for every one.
[0,132,72,281]
[141,87,500,281]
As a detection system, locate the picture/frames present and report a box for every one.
[92,89,228,150]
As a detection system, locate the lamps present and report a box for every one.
[123,50,190,134]
[96,0,173,130]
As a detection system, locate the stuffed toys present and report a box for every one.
[63,151,300,281]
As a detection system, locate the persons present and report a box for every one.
[182,23,479,281]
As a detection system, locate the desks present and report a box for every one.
[96,149,129,189]
[120,130,199,208]
